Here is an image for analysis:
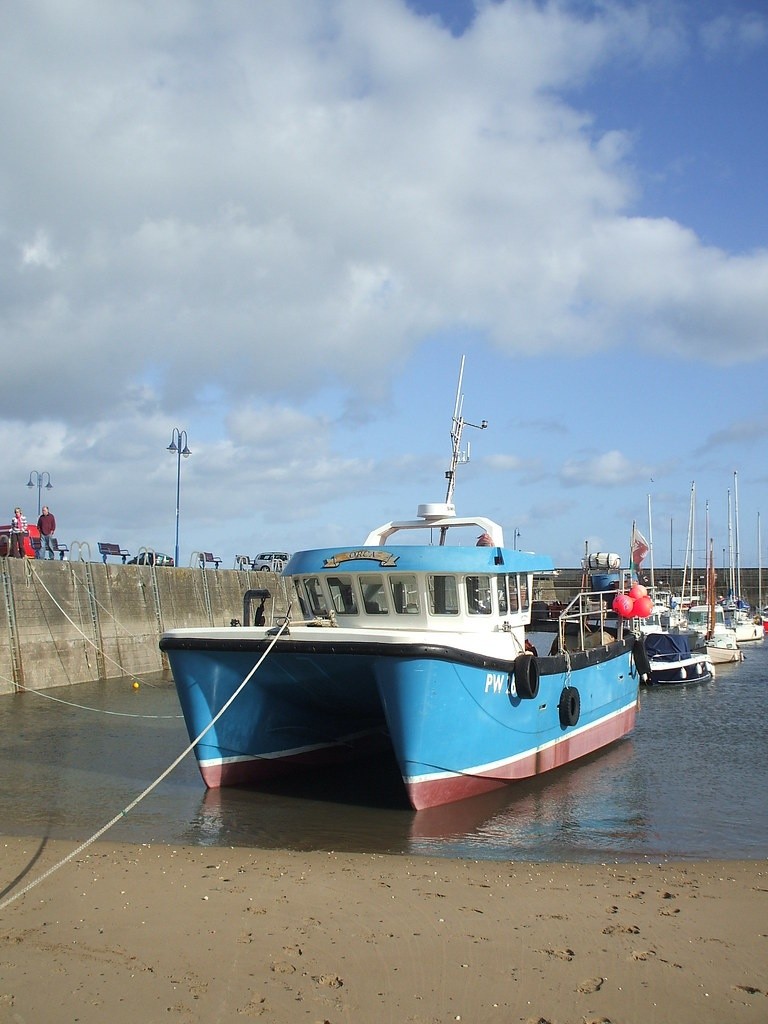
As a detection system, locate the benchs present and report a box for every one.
[29,537,69,561]
[191,552,222,569]
[236,554,258,570]
[98,542,130,564]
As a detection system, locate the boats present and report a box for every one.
[158,353,644,812]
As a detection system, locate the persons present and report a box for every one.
[11,507,28,558]
[37,506,56,560]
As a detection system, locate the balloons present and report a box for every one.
[613,585,653,618]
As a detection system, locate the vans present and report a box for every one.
[251,551,292,572]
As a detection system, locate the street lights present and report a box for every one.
[26,470,53,517]
[167,427,193,566]
[513,526,522,549]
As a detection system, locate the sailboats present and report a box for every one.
[552,471,768,686]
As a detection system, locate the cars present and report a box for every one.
[128,551,173,567]
